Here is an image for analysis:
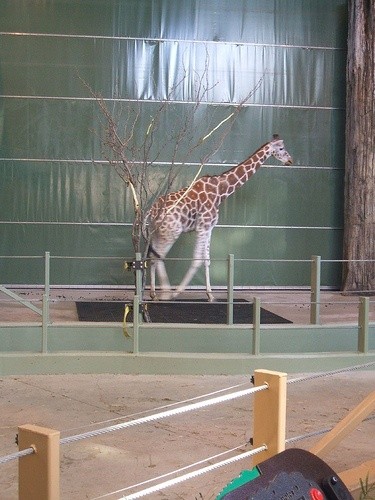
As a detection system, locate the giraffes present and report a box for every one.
[141,133,293,303]
